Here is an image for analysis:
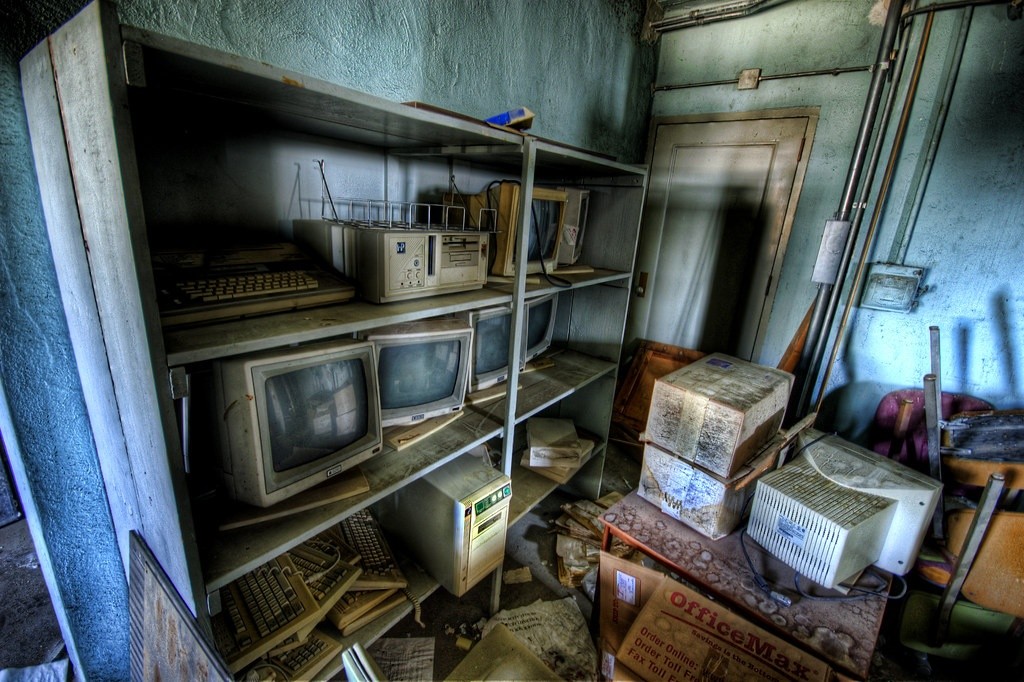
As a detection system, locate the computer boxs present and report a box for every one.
[350,224,486,304]
[378,452,512,598]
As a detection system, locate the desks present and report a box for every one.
[592,487,893,682]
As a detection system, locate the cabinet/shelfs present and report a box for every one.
[20,0,648,682]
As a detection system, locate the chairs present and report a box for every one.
[873,326,1024,682]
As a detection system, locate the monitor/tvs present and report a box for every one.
[747,428,945,596]
[452,306,528,404]
[523,291,554,374]
[469,182,594,283]
[182,339,382,530]
[364,318,474,452]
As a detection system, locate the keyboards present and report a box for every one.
[214,507,408,682]
[159,269,355,324]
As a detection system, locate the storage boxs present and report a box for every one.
[598,549,844,682]
[637,351,796,540]
